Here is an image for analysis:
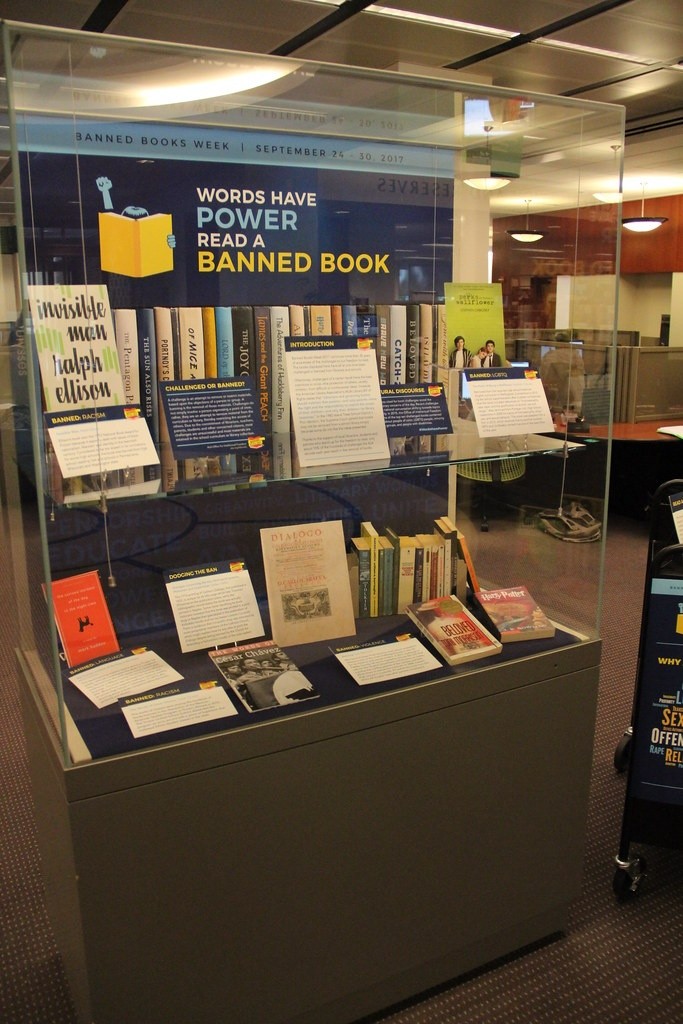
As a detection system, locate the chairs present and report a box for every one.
[454,455,532,531]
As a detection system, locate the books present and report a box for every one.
[13,265,571,782]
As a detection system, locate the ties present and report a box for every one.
[489,355,492,366]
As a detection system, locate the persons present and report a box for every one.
[480,340,502,368]
[555,331,571,350]
[449,336,473,368]
[469,346,490,369]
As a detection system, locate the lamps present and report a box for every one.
[452,122,511,194]
[506,197,546,241]
[622,178,668,233]
